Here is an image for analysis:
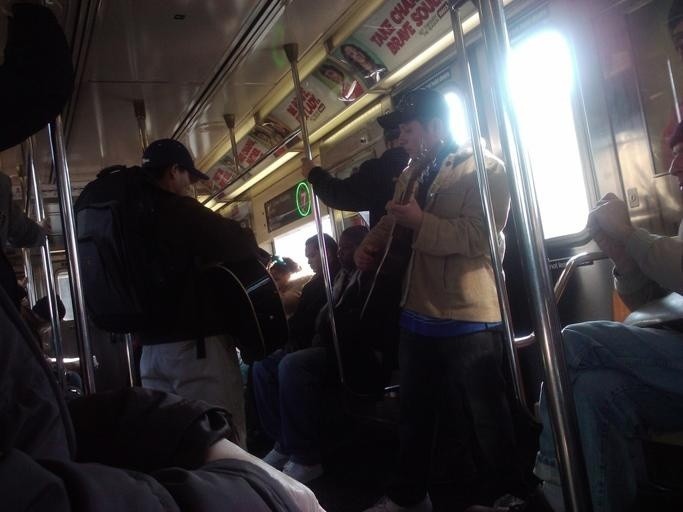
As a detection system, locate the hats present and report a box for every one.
[375,88,440,132]
[33,294,66,323]
[1,2,75,152]
[141,138,210,185]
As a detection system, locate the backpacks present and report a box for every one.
[70,163,167,336]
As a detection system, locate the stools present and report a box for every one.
[523,385,683,509]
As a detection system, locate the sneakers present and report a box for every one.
[362,486,434,512]
[281,454,326,484]
[466,492,531,512]
[261,449,290,470]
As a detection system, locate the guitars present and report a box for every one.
[331,140,446,420]
[198,259,289,353]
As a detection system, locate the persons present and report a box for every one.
[533,125,683,512]
[659,0,683,173]
[1,0,532,512]
